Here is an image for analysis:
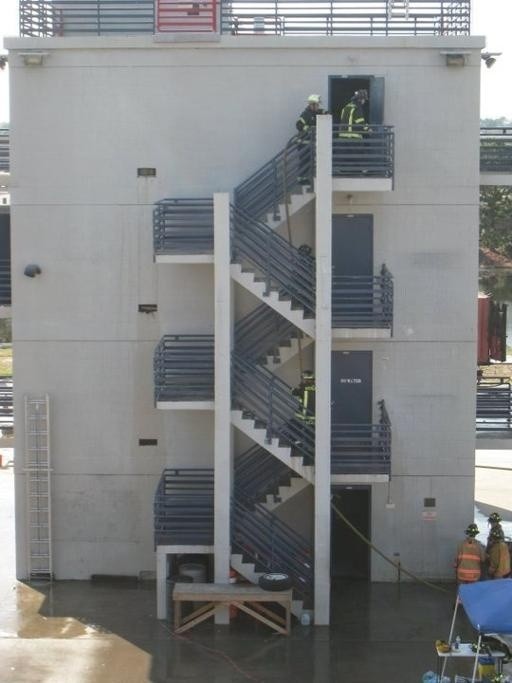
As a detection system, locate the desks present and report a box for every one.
[437,643,506,683]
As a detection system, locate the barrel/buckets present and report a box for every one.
[180,563,208,610]
[165,575,194,621]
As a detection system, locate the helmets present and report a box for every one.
[350,90,369,100]
[487,528,505,539]
[307,94,320,103]
[488,512,503,522]
[300,370,314,380]
[464,523,480,536]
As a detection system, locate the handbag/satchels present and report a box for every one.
[472,635,511,663]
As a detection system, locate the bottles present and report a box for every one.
[455,635,461,650]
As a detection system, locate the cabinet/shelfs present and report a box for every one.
[174,583,292,636]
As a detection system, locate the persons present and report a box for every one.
[487,526,511,579]
[290,368,314,454]
[296,93,329,186]
[336,88,375,175]
[486,512,505,551]
[453,522,487,587]
